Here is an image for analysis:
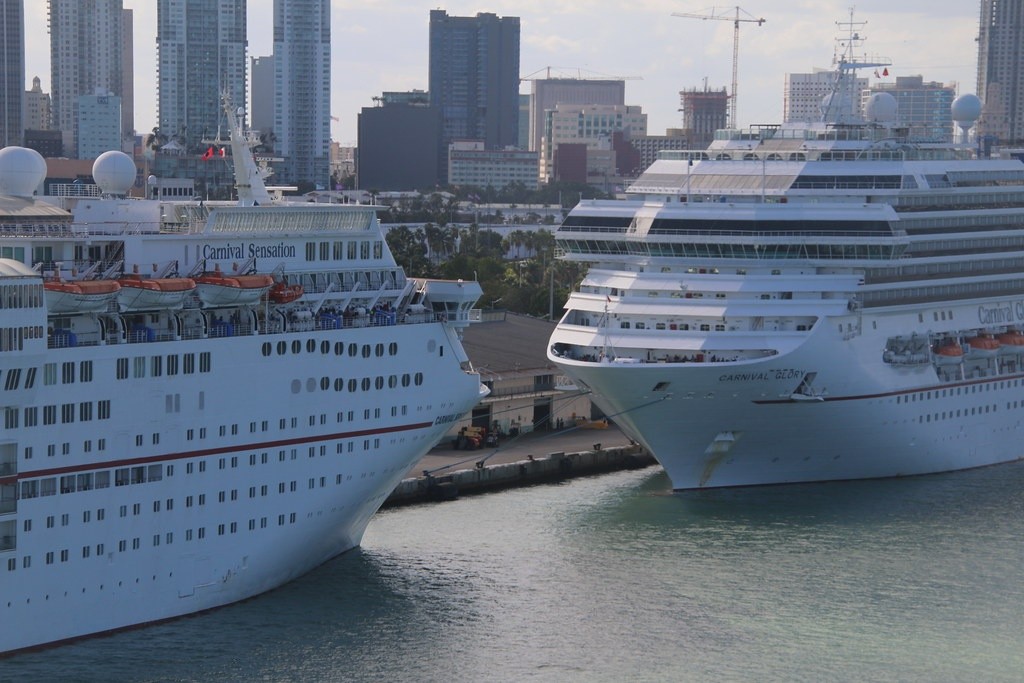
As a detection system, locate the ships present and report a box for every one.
[0,188,491,660]
[547,92,1024,494]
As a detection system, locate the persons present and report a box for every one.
[318,305,384,315]
[556,417,564,431]
[210,314,234,325]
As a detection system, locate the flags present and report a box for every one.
[882,68,889,77]
[607,295,612,303]
[874,69,880,78]
[218,146,225,159]
[202,147,213,161]
